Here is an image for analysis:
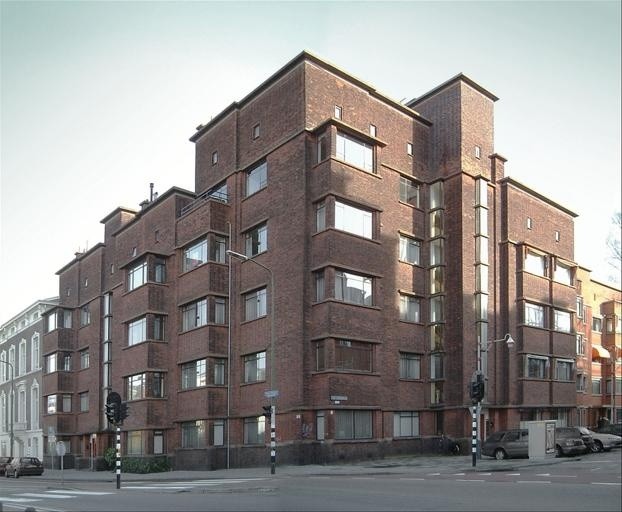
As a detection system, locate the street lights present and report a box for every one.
[226,250,276,477]
[472,332,517,460]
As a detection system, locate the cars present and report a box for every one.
[0,456,44,478]
[482,423,622,460]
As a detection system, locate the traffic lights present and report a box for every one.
[104,392,130,425]
[470,382,485,406]
